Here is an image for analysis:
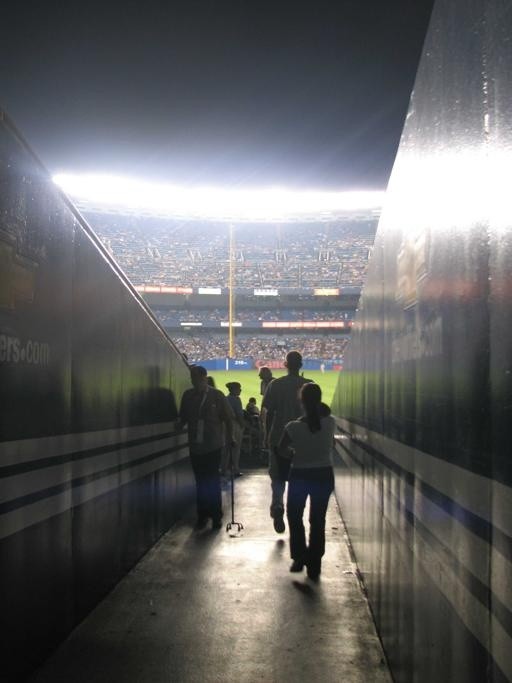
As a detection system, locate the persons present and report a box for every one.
[276,382,335,578]
[260,351,314,533]
[83,211,375,287]
[151,304,355,360]
[174,365,236,530]
[320,362,325,374]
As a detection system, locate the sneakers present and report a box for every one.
[290,548,321,579]
[195,516,223,530]
[273,507,285,533]
